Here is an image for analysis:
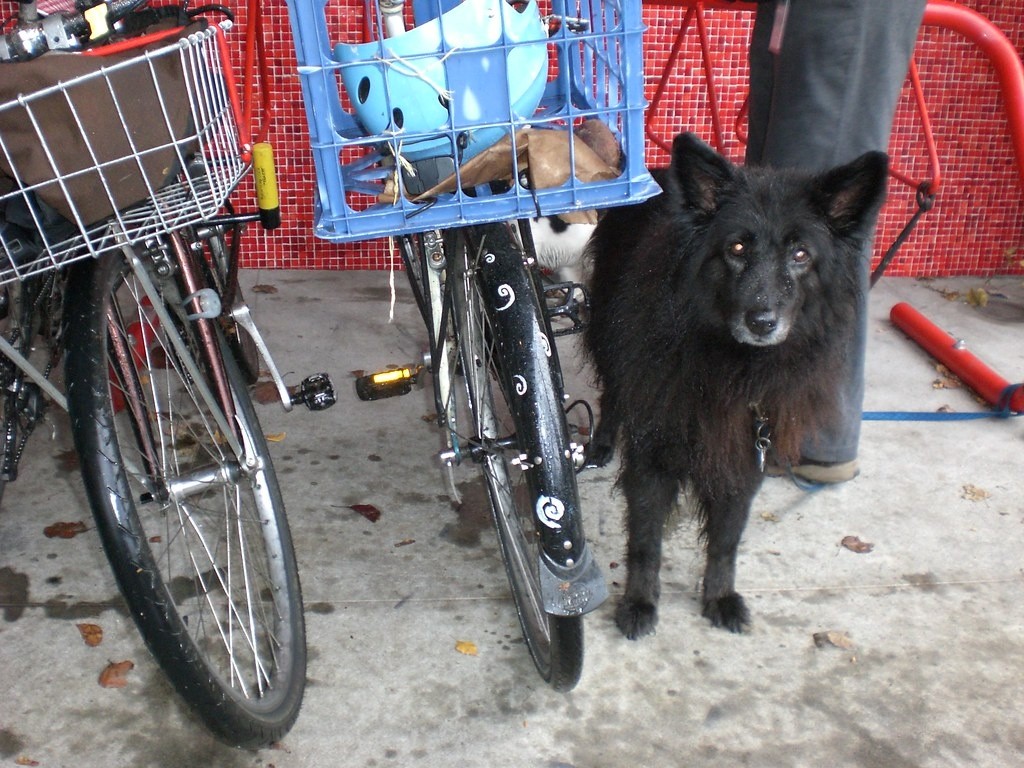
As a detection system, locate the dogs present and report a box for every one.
[574,131,889,640]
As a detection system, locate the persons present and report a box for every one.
[744,0,930,487]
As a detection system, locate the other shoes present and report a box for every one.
[763,455,860,482]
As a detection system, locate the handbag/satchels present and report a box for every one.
[0,3,206,230]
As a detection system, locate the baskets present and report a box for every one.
[0,21,254,284]
[285,0,663,244]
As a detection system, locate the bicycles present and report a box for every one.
[281,1,664,695]
[0,1,341,751]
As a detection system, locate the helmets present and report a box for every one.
[333,0,548,194]
